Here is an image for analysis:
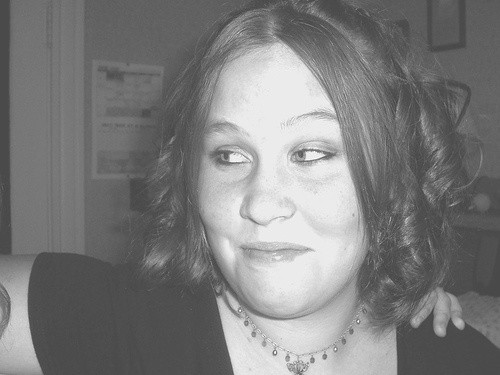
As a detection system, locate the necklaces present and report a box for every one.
[233,282,369,374]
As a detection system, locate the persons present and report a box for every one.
[0,2,500,375]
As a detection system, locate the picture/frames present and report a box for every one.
[427,0,466,52]
